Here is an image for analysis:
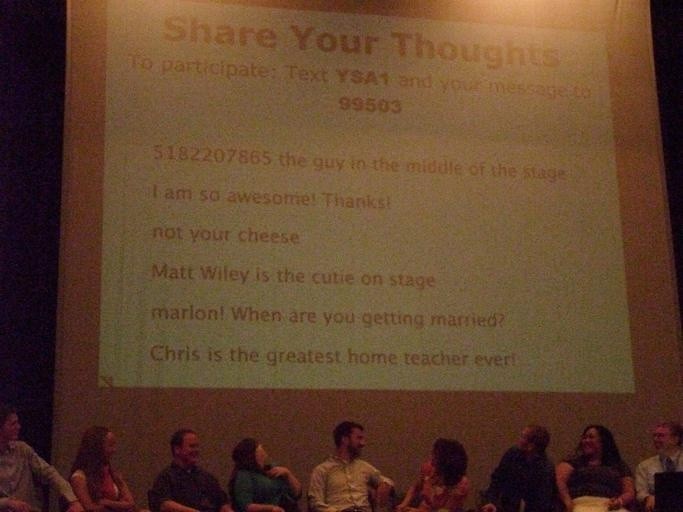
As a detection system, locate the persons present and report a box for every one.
[557,425,636,512]
[397,438,469,511]
[0,406,84,512]
[634,419,683,511]
[307,420,396,512]
[61,424,134,511]
[231,438,303,512]
[480,424,559,511]
[151,428,233,512]
